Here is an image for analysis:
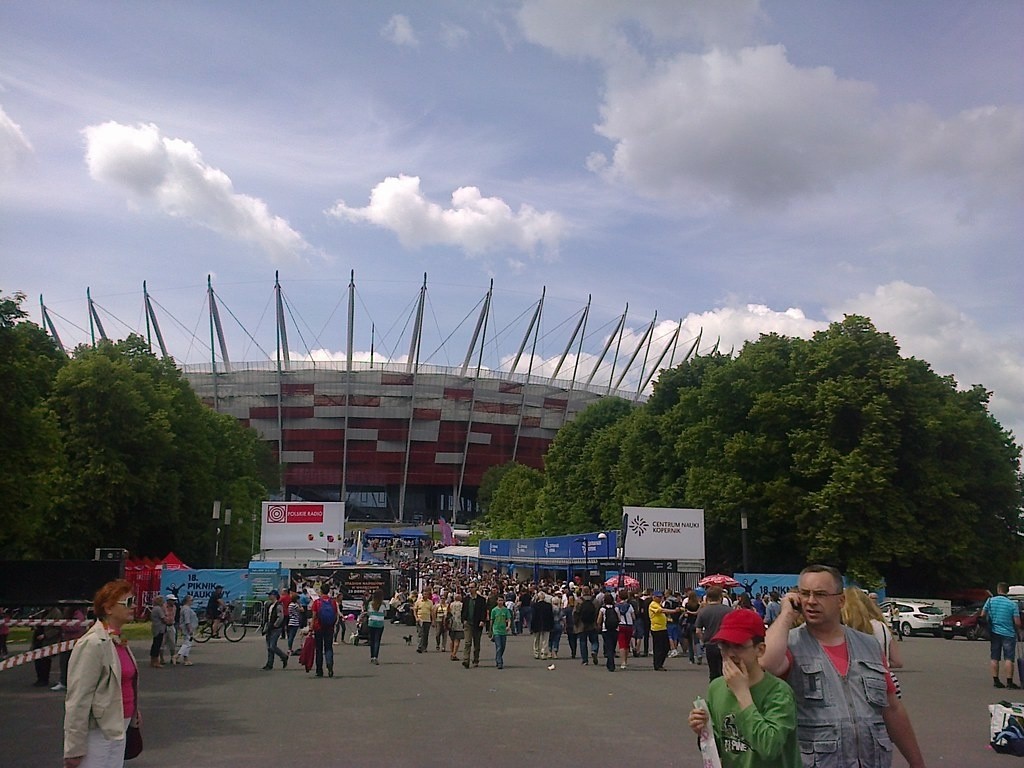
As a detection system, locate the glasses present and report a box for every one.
[717,641,756,651]
[796,590,843,600]
[467,586,475,589]
[116,596,136,608]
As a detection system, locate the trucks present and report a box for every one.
[1007,585,1024,613]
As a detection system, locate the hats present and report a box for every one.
[166,594,177,601]
[265,590,278,595]
[710,609,766,643]
[652,591,663,597]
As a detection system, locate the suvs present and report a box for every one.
[879,600,948,637]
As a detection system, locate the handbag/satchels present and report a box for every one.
[974,596,992,640]
[125,714,142,760]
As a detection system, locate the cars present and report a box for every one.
[940,605,991,641]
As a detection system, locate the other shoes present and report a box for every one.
[548,654,559,659]
[50,682,65,690]
[259,665,273,671]
[369,655,379,664]
[416,647,503,669]
[581,648,703,672]
[287,649,294,657]
[151,656,193,668]
[315,664,334,678]
[282,655,289,668]
[211,635,221,639]
[280,637,285,640]
[993,678,1021,689]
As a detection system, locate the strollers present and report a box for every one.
[353,612,370,646]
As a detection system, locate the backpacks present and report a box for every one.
[316,597,336,627]
[579,597,597,626]
[601,605,619,631]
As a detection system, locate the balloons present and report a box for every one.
[348,614,355,621]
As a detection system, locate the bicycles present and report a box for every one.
[191,609,247,643]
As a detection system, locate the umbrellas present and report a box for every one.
[604,575,640,588]
[698,573,739,588]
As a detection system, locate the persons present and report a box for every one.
[150,594,198,667]
[31,601,86,690]
[369,537,903,670]
[688,608,802,768]
[205,585,231,639]
[0,608,10,658]
[979,583,1021,689]
[259,584,348,677]
[361,590,387,665]
[759,564,928,768]
[63,581,143,768]
[291,570,336,594]
[344,529,365,547]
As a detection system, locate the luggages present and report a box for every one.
[1015,634,1024,689]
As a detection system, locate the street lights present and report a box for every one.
[598,531,610,562]
[212,499,221,568]
[741,512,749,573]
[223,508,232,569]
[573,538,588,568]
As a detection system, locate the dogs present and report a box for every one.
[349,632,360,646]
[403,634,413,646]
